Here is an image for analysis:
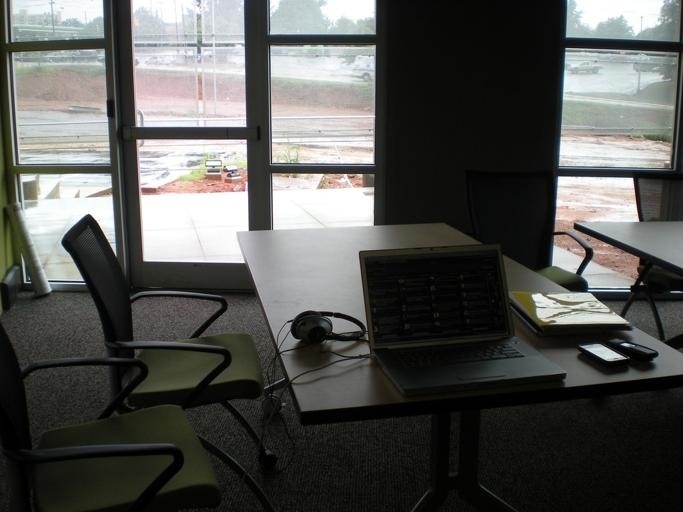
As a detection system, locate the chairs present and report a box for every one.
[617,168,683,351]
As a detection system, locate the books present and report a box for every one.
[508,290,630,330]
[509,300,633,338]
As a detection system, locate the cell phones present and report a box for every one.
[576,340,631,371]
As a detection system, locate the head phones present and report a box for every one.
[290,309,367,345]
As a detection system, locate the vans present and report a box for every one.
[350,55,376,81]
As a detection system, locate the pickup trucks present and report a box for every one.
[569,61,602,73]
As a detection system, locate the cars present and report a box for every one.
[15,48,229,67]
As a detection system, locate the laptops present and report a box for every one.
[357,241,568,397]
